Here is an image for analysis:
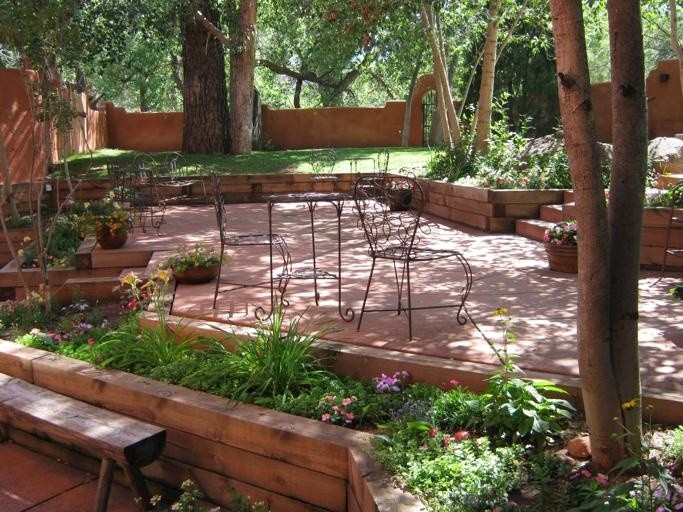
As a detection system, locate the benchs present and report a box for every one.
[0,372,167,512]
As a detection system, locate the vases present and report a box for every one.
[95,229,128,250]
[174,266,218,284]
[546,242,577,272]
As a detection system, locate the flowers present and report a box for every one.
[91,206,132,236]
[155,240,231,275]
[541,219,581,245]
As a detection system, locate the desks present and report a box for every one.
[255,193,358,322]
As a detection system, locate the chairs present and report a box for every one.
[354,173,473,339]
[209,172,293,309]
[106,149,208,238]
